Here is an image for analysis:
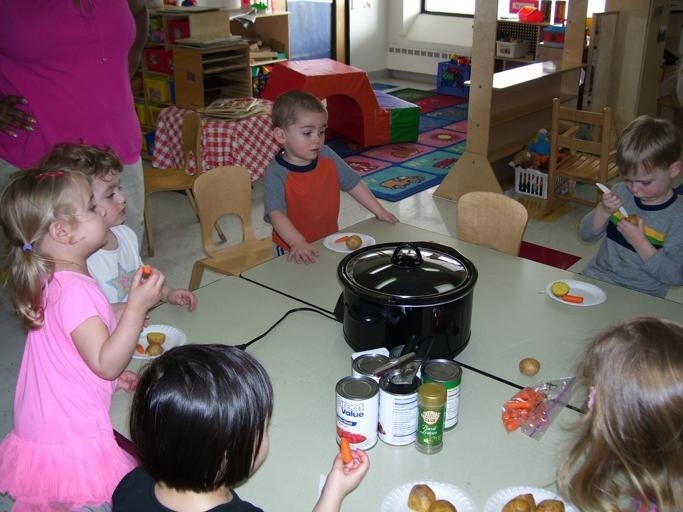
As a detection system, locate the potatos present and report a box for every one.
[407,484,436,511]
[144,342,164,356]
[519,357,541,376]
[551,280,570,297]
[502,492,566,511]
[429,499,458,511]
[147,332,165,345]
[346,234,362,250]
[625,213,640,227]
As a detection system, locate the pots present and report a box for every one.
[336,240,478,364]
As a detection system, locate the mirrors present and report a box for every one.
[587,14,617,113]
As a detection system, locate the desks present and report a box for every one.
[152,99,283,188]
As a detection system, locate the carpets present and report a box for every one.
[325,88,468,202]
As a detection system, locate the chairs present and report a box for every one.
[548,97,620,213]
[189,167,276,291]
[144,113,227,256]
[456,192,528,257]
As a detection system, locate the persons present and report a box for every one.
[0,165,166,512]
[551,316,683,512]
[0,0,145,256]
[576,114,683,300]
[36,142,198,334]
[262,90,401,266]
[110,342,370,512]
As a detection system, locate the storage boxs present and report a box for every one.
[131,19,189,155]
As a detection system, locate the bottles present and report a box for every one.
[414,383,446,453]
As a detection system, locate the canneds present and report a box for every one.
[335,352,464,456]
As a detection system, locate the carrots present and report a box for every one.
[143,265,152,275]
[136,345,144,353]
[340,437,353,465]
[564,292,587,304]
[501,387,543,432]
[336,234,350,243]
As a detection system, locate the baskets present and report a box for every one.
[509,162,568,199]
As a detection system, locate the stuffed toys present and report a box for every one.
[440,53,469,88]
[511,127,570,195]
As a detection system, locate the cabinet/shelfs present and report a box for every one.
[172,43,253,107]
[494,19,549,72]
[229,0,290,67]
[588,0,683,138]
[133,11,229,161]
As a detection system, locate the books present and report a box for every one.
[205,96,265,121]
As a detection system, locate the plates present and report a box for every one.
[476,483,578,512]
[128,323,188,360]
[379,479,479,512]
[545,277,607,310]
[321,232,376,256]
